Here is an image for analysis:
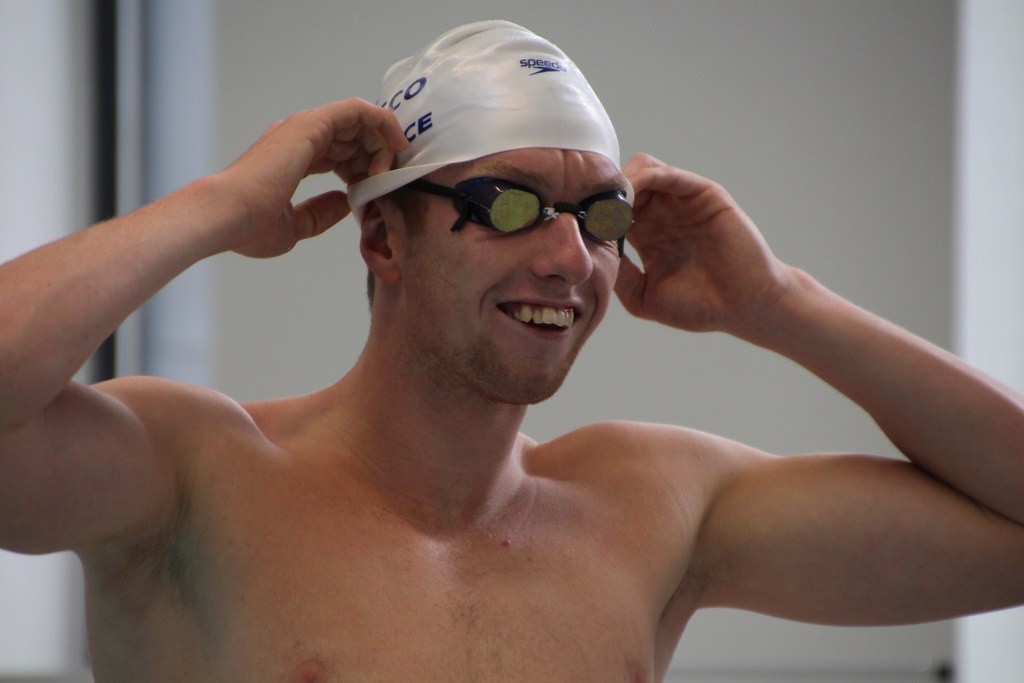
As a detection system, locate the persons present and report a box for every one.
[1,18,1023,681]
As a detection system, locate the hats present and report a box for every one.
[347,18,645,274]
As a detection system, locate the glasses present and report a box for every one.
[418,178,636,259]
